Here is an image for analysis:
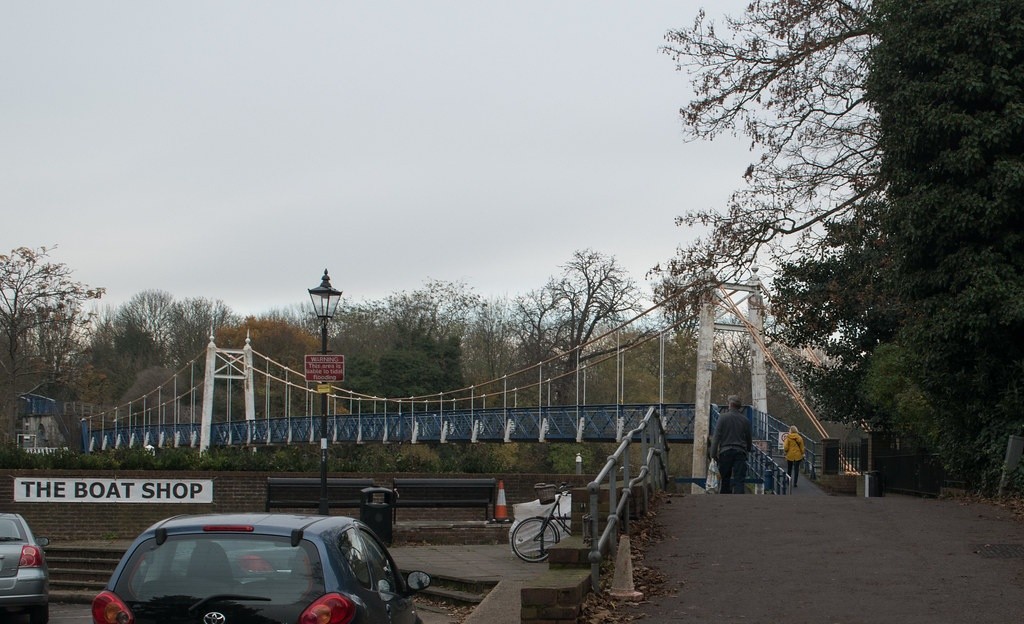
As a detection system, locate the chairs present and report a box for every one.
[296,546,313,597]
[190,542,242,589]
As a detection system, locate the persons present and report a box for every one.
[710,395,752,494]
[784,425,805,488]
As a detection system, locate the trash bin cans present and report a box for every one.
[821,437,841,476]
[359,485,394,547]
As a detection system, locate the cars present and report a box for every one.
[0,512,51,624]
[90,513,432,624]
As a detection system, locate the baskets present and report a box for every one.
[534,483,556,505]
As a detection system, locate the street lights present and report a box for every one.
[306,267,344,514]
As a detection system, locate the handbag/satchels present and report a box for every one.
[705,458,722,494]
[800,458,805,469]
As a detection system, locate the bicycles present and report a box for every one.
[511,482,578,564]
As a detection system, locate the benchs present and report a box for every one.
[266,477,374,513]
[392,478,495,524]
